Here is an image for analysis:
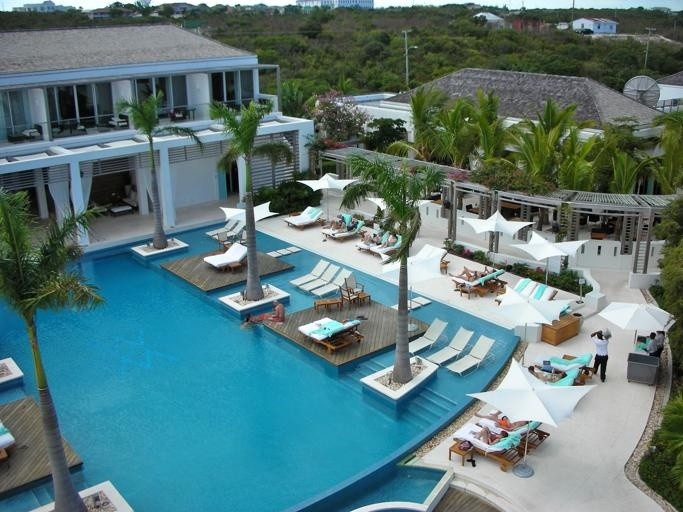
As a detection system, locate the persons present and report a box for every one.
[267,299,284,322]
[463,266,496,276]
[359,230,380,244]
[469,426,508,446]
[530,363,566,382]
[473,409,530,428]
[239,318,251,330]
[322,219,341,229]
[447,271,478,282]
[378,232,399,249]
[336,213,346,232]
[460,282,478,294]
[247,311,273,322]
[646,331,665,357]
[589,330,609,383]
[643,332,658,358]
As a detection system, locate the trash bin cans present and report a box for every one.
[627,353,659,386]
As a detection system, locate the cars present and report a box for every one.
[576,28,594,35]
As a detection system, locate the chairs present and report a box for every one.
[8,106,197,144]
[405,318,498,377]
[434,249,561,319]
[525,351,594,404]
[204,205,251,274]
[0,417,15,460]
[279,258,373,355]
[88,185,137,217]
[284,205,408,262]
[446,405,553,470]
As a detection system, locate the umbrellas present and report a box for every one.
[594,301,680,345]
[292,172,359,221]
[493,286,572,366]
[457,208,537,251]
[217,198,279,229]
[464,354,597,469]
[509,229,590,285]
[365,195,434,213]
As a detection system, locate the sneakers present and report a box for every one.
[467,459,476,466]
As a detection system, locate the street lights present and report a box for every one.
[405,45,419,87]
